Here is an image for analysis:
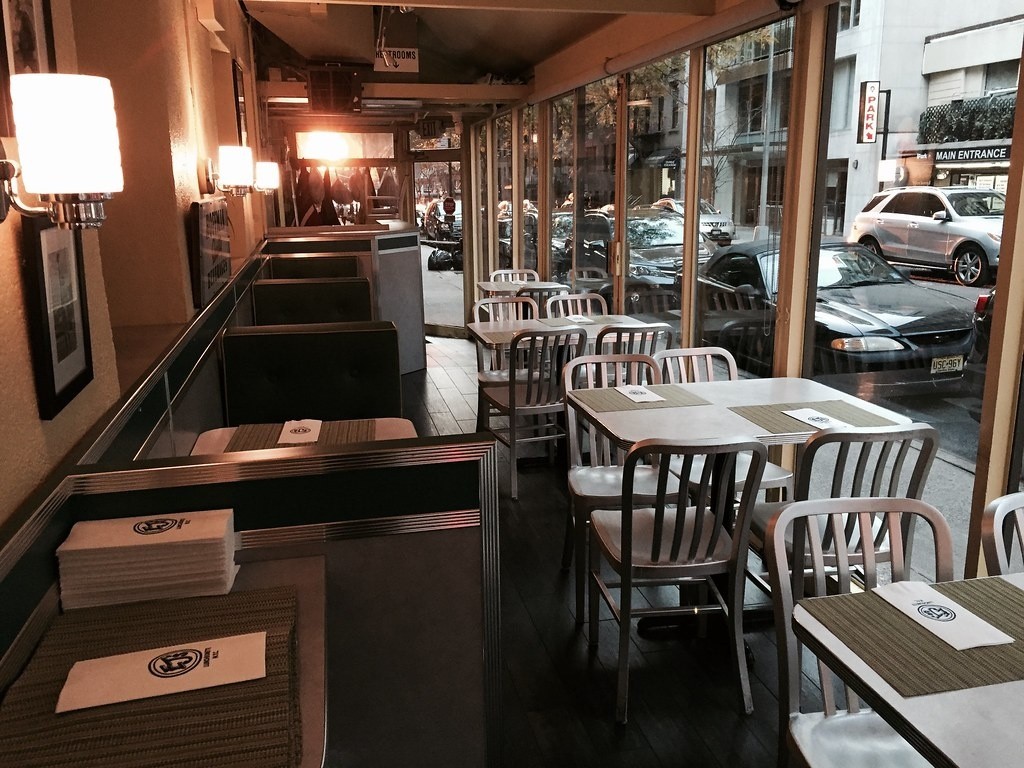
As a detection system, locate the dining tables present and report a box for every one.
[468,314,666,371]
[568,377,914,640]
[792,571,1024,768]
[476,281,571,318]
[188,417,419,456]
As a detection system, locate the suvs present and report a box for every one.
[650,198,740,245]
[564,210,717,314]
[847,185,1006,286]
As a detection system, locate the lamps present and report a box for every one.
[224,161,282,199]
[0,73,125,230]
[198,144,260,195]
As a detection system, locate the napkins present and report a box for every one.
[56,631,266,715]
[613,385,667,404]
[871,580,1016,651]
[55,508,242,613]
[781,407,856,431]
[277,419,322,442]
[565,315,594,323]
[510,280,527,284]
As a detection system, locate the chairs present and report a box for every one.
[646,346,793,535]
[473,269,609,411]
[733,423,940,712]
[767,495,955,768]
[588,437,755,724]
[577,322,677,456]
[478,325,588,502]
[980,491,1024,577]
[561,354,690,632]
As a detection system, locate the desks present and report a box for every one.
[0,555,327,768]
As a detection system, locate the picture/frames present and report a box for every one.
[21,213,96,422]
[1,0,59,73]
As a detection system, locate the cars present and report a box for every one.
[974,289,997,363]
[674,234,976,398]
[415,198,573,271]
